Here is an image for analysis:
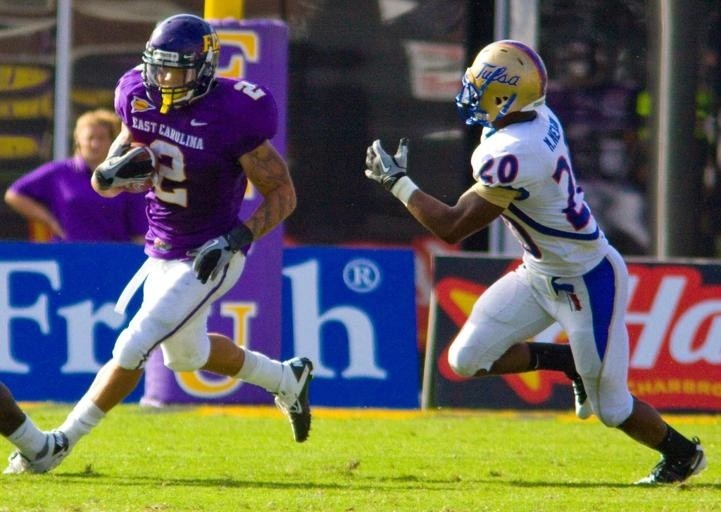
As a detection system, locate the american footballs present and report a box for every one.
[115,145,161,193]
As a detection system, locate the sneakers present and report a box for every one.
[571,376,593,421]
[274,357,313,442]
[635,439,706,489]
[4,429,69,475]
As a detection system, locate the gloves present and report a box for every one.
[93,145,154,190]
[185,229,243,284]
[365,136,410,194]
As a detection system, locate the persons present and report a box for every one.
[4,14,314,475]
[3,109,150,244]
[0,381,74,473]
[363,39,708,487]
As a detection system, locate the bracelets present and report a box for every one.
[224,223,254,251]
[388,175,419,208]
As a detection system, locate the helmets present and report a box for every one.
[454,39,548,126]
[141,14,219,113]
